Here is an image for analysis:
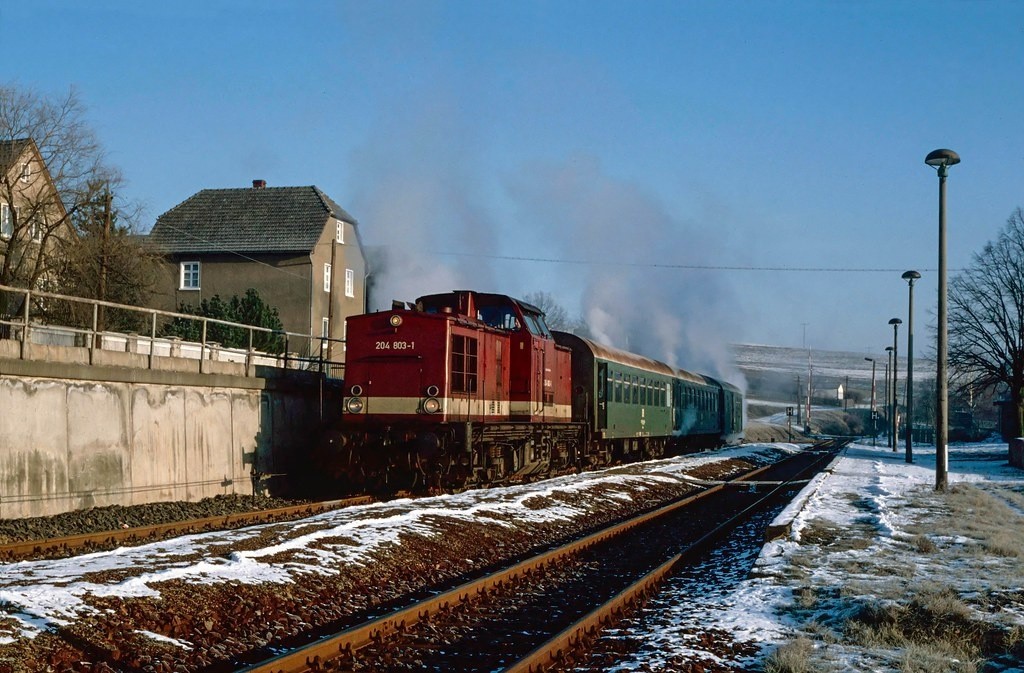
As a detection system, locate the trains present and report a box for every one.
[323,289,743,497]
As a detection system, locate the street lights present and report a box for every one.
[886,346,893,447]
[901,270,921,463]
[889,318,903,453]
[865,358,876,447]
[926,150,963,488]
